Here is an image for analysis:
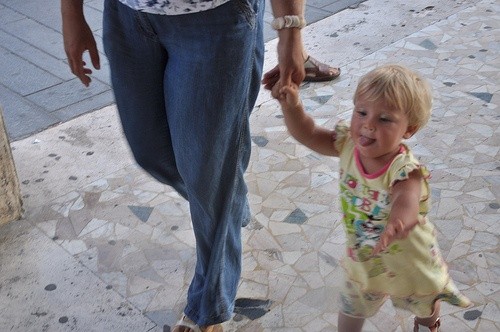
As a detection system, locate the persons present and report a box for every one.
[59,0,307,331]
[271,63,472,332]
[302,42,340,83]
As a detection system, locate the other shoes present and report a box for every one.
[413,297,441,332]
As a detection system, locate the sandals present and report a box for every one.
[172,311,215,332]
[300,56,341,81]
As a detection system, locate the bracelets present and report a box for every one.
[270,14,306,30]
